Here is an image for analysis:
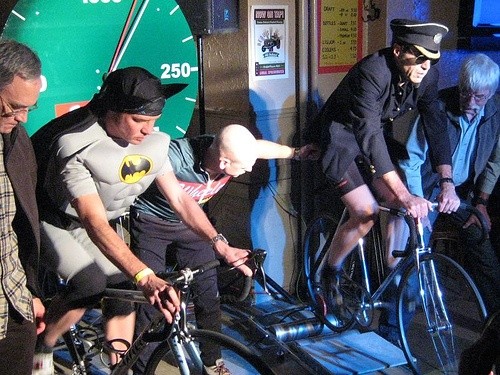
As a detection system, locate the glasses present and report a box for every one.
[1,95,40,117]
[458,87,491,102]
[404,43,440,66]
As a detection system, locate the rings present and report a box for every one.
[449,208,454,211]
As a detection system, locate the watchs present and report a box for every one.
[294,147,301,161]
[210,233,228,251]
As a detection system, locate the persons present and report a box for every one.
[0,40,49,375]
[28,66,257,375]
[306,18,460,314]
[377,52,500,351]
[128,124,321,375]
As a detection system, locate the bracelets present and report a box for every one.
[134,267,154,286]
[439,178,454,188]
[473,197,489,206]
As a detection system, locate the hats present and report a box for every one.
[389,17,449,59]
[99,65,189,116]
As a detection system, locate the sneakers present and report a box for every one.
[320,266,348,308]
[379,332,418,368]
[204,358,233,375]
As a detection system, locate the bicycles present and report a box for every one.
[303,159,490,375]
[38,250,275,375]
